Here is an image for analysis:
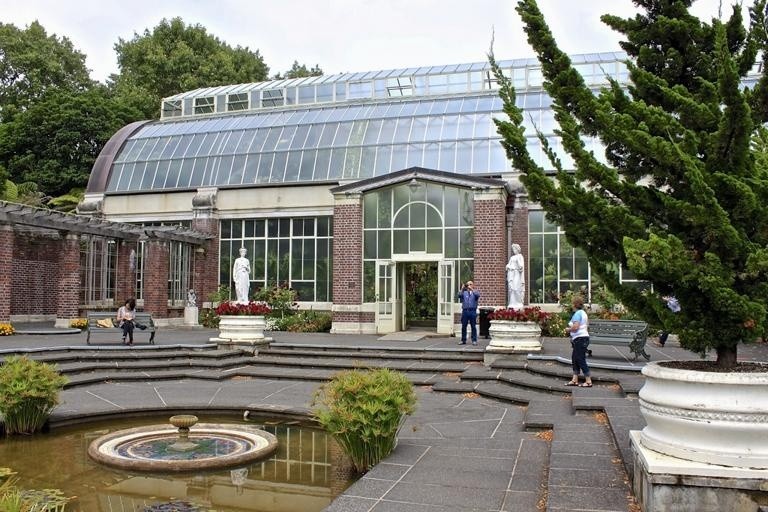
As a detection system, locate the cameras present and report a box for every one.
[464,284,468,288]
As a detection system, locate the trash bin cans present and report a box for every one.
[478,306,495,336]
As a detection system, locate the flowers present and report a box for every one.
[212,299,271,318]
[486,305,549,322]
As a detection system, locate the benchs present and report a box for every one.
[86,312,160,344]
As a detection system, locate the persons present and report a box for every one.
[188,289,196,306]
[653,291,680,347]
[233,247,251,307]
[458,281,480,345]
[116,298,136,345]
[564,297,592,387]
[506,244,526,308]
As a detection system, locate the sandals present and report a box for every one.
[578,382,593,387]
[564,380,579,386]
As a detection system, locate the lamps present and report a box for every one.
[406,178,423,194]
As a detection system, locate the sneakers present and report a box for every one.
[472,341,478,346]
[458,341,466,345]
